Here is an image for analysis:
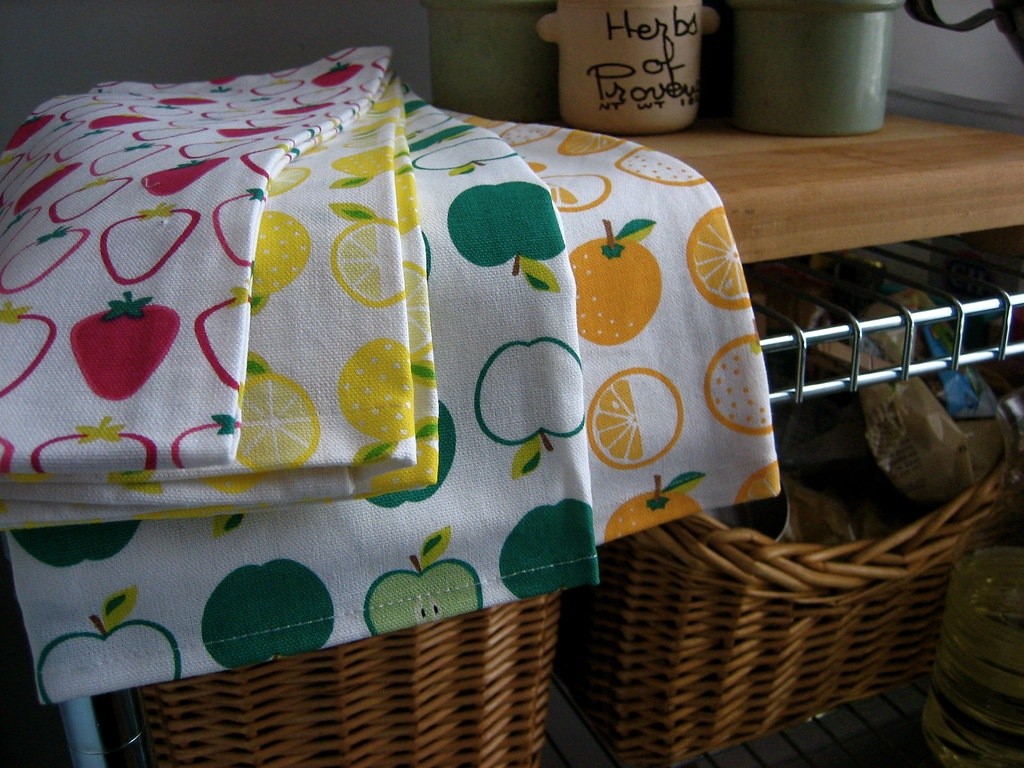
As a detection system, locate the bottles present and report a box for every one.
[923,389,1024,768]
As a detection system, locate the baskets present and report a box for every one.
[112,589,564,768]
[574,359,1024,768]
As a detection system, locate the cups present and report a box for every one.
[419,0,552,124]
[724,0,905,135]
[536,0,720,130]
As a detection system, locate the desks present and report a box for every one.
[64,117,1023,767]
